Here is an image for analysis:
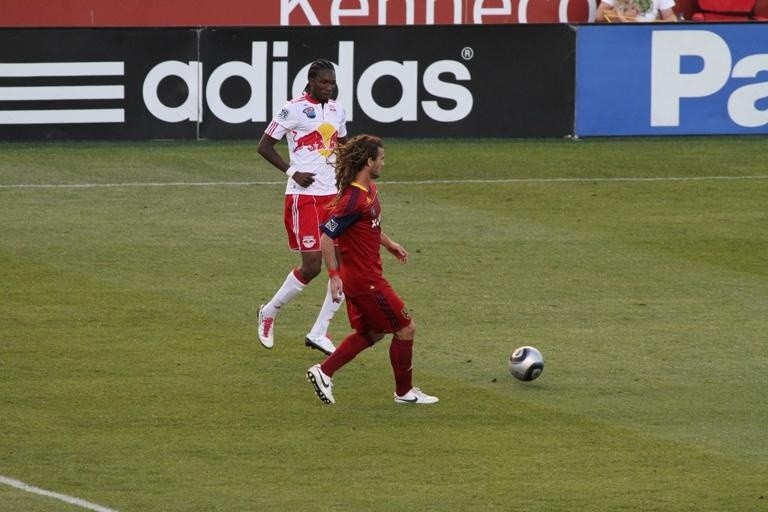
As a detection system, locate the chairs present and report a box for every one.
[692,0,768,21]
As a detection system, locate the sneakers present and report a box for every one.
[307,364,335,405]
[393,387,439,404]
[304,333,337,355]
[258,304,274,349]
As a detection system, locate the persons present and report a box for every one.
[594,0,678,23]
[256,58,350,355]
[307,134,440,404]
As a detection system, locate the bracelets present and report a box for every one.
[328,269,341,278]
[286,166,297,178]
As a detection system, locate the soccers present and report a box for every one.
[508,346,543,380]
